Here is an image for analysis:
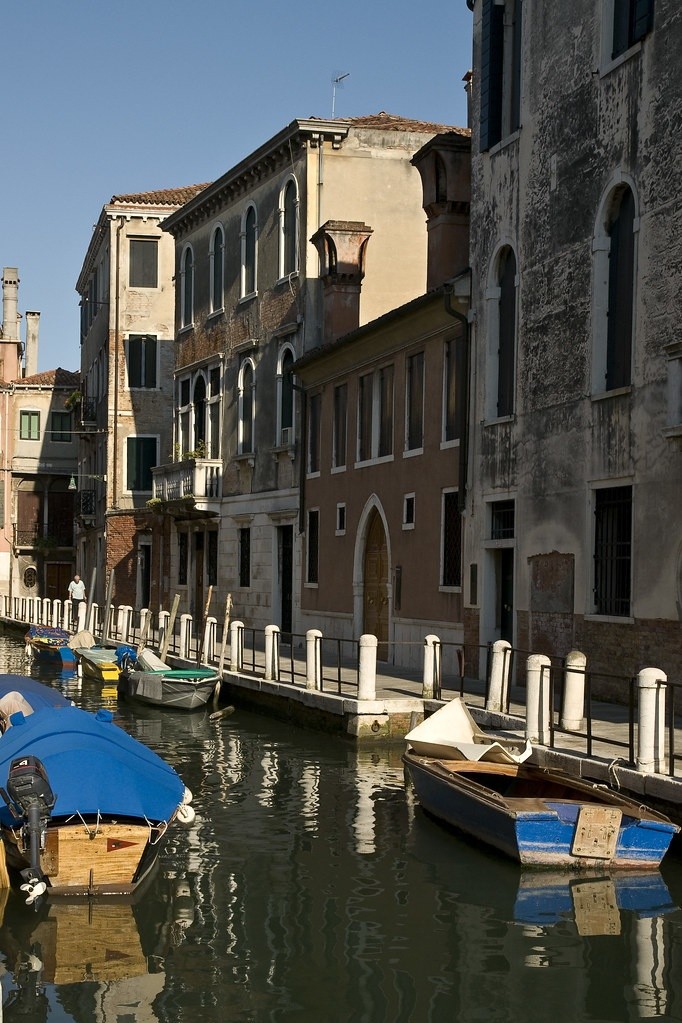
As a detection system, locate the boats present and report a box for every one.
[0,674,185,896]
[401,697,682,869]
[25,626,123,681]
[397,808,682,936]
[112,645,222,710]
[0,897,174,1023]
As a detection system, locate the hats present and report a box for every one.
[74,575,80,579]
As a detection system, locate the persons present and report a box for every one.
[68,574,87,622]
